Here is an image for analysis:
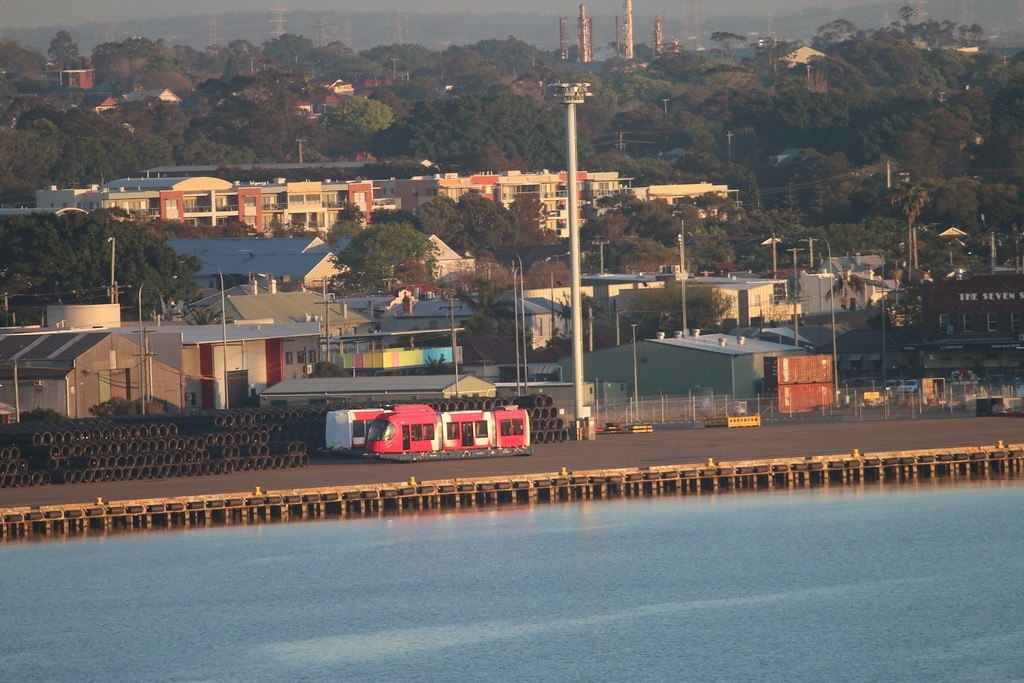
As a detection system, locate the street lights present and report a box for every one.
[546,79,593,438]
[106,237,115,304]
[179,259,230,410]
[630,323,639,423]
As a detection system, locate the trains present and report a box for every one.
[364,404,533,462]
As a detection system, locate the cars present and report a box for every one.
[879,380,900,396]
[839,377,876,388]
[896,380,917,394]
